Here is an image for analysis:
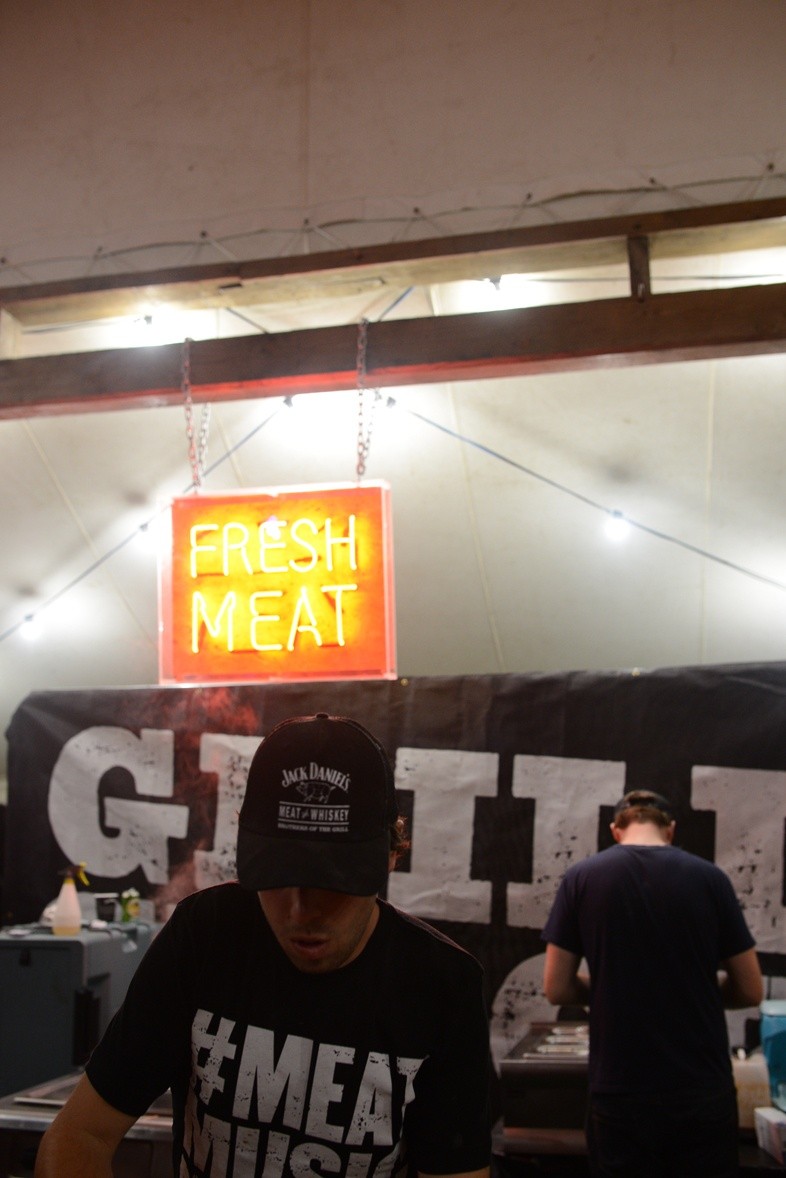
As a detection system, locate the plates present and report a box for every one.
[539,1026,590,1057]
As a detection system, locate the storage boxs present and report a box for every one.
[753,1105,785,1165]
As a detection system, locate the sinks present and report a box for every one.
[14,1071,173,1114]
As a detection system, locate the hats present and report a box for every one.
[235,710,398,893]
[618,790,673,819]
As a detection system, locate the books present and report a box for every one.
[521,1023,589,1059]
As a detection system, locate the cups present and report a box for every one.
[122,891,141,921]
[95,892,116,923]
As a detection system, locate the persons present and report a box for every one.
[542,790,763,1178]
[34,712,495,1178]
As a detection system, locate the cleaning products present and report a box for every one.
[52,861,89,934]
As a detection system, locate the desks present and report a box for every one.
[500,1020,591,1178]
[0,917,163,1094]
